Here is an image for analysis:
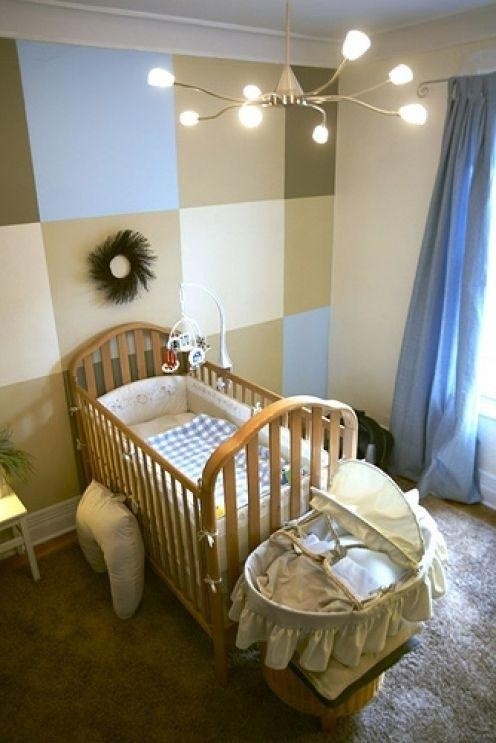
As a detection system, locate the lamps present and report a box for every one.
[147,0,428,144]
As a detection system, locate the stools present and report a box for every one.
[261,638,384,732]
[0,471,40,583]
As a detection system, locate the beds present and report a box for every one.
[66,321,359,675]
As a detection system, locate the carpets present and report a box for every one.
[0,484,496,743]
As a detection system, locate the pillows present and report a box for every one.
[76,478,146,619]
